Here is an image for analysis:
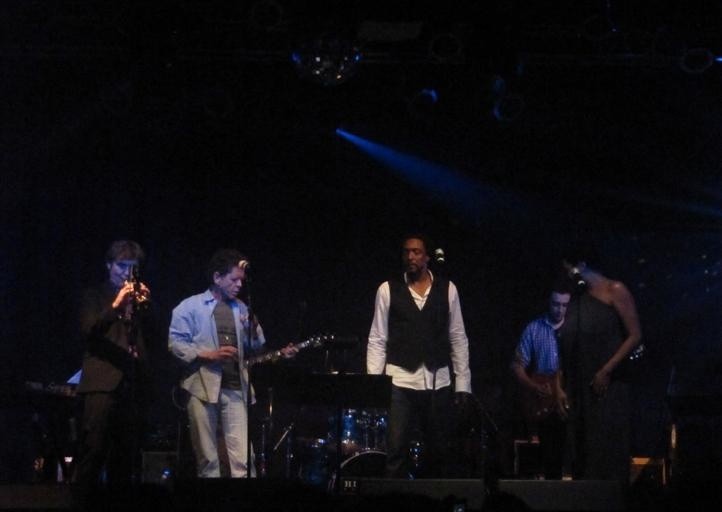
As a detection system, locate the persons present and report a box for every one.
[553,248,644,482]
[72,239,152,484]
[364,235,474,479]
[511,291,581,482]
[167,250,301,480]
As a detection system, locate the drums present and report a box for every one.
[330,411,387,452]
[327,450,415,499]
[287,406,331,459]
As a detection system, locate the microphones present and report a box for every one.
[570,265,586,287]
[237,260,256,275]
[434,248,446,265]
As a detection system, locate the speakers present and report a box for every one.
[494,479,631,512]
[352,479,489,512]
[166,478,257,512]
[0,482,77,512]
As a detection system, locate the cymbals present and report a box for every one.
[313,370,363,376]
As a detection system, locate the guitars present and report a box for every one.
[514,368,575,434]
[172,333,336,409]
